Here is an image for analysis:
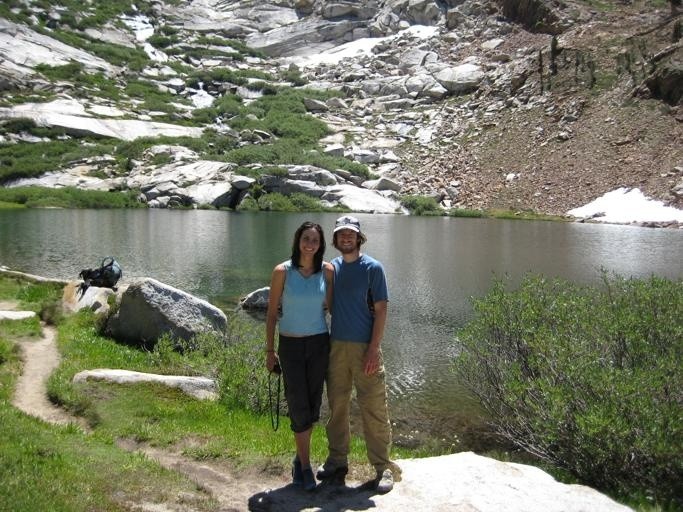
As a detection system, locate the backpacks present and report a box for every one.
[79,255,123,288]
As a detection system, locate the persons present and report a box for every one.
[278,216,393,492]
[266,221,374,491]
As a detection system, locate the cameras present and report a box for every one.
[273,364,282,375]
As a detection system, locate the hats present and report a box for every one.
[332,215,361,234]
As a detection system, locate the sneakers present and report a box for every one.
[315,462,349,483]
[298,466,317,492]
[372,467,396,494]
[290,457,305,489]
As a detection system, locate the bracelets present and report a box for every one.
[267,350,275,352]
